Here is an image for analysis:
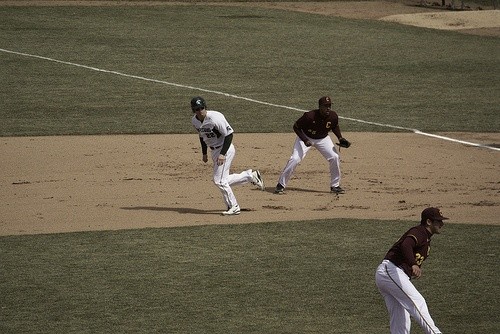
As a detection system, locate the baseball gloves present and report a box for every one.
[335,137,351,149]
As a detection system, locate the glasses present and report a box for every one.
[195,107,204,111]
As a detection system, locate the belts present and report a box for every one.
[210,146,220,149]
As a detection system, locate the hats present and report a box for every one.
[421,207,449,221]
[318,96,334,106]
[190,97,206,109]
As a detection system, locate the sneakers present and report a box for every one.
[252,169,264,190]
[222,205,240,215]
[275,184,283,193]
[331,185,344,192]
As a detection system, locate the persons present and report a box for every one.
[190,96,263,215]
[376,207,450,334]
[275,96,352,193]
